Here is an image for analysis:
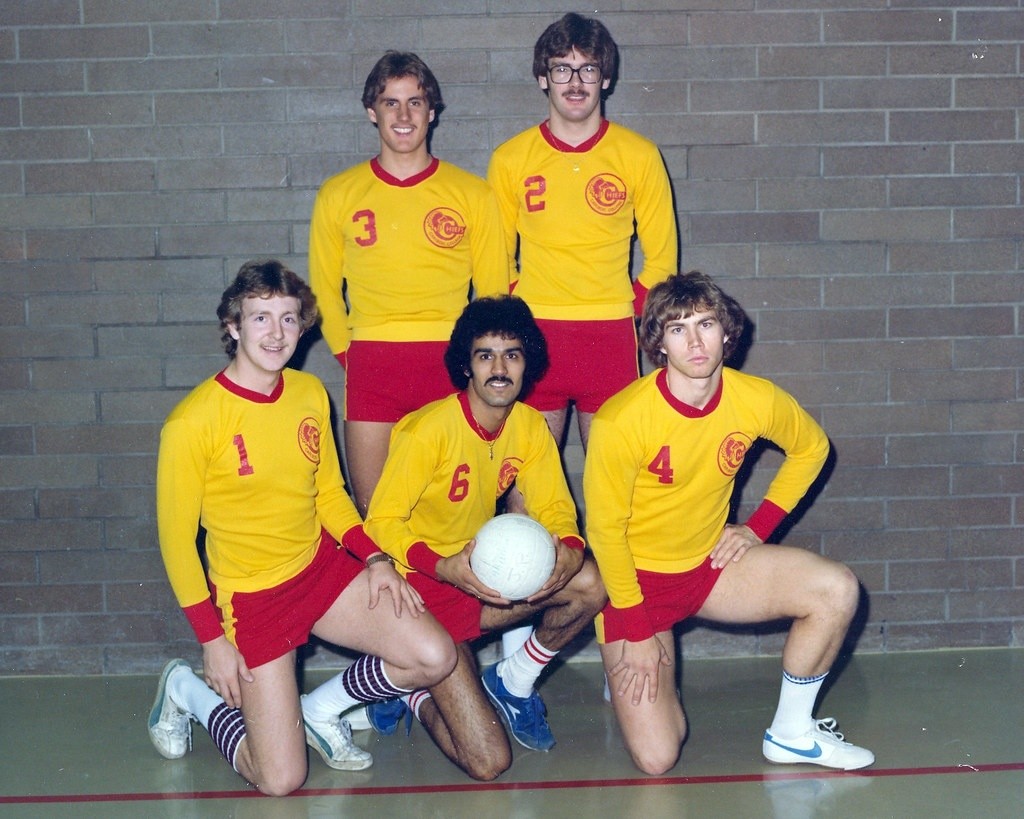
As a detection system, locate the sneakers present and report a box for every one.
[365,696,414,737]
[299,694,374,771]
[148,658,199,760]
[480,658,557,753]
[763,718,874,771]
[340,703,372,731]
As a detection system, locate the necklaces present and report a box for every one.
[472,402,514,460]
[549,124,602,171]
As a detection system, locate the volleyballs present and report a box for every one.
[469,512,556,601]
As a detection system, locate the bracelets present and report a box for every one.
[367,554,392,568]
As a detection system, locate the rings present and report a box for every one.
[478,596,480,598]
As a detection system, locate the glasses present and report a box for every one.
[546,64,603,84]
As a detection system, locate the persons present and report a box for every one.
[342,295,608,782]
[583,270,874,775]
[310,52,510,518]
[146,260,458,797]
[487,11,678,658]
